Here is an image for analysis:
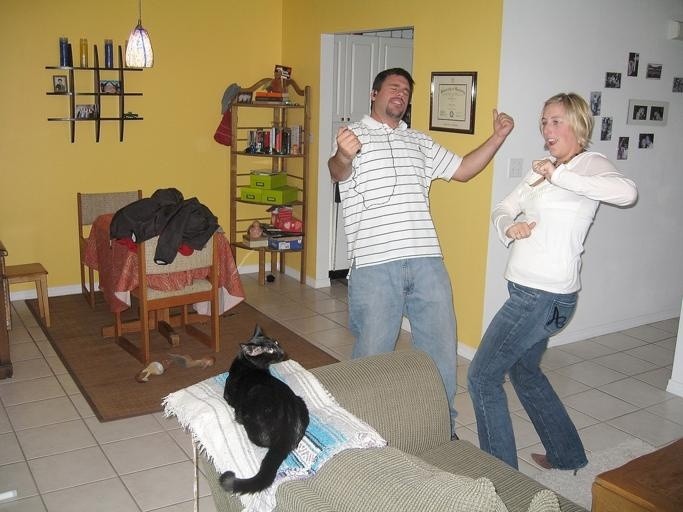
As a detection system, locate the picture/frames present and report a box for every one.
[429,72,477,134]
[53,75,68,93]
[627,99,669,127]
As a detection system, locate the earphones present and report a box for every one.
[373,94,376,97]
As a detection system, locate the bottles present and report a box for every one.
[59,39,69,66]
[250,138,264,153]
[105,40,112,68]
[79,39,88,68]
[248,220,261,239]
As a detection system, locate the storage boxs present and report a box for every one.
[262,185,297,205]
[250,171,287,189]
[269,234,303,251]
[240,186,262,203]
[242,234,268,248]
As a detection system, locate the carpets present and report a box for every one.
[23,290,339,423]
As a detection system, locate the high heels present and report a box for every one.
[531,453,579,475]
[174,354,214,370]
[135,360,168,383]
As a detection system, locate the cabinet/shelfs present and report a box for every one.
[229,76,312,286]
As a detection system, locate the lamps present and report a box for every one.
[124,1,154,68]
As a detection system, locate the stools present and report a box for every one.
[6,263,51,331]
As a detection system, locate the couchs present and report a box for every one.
[199,350,590,512]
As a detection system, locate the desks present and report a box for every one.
[0,243,15,379]
[85,212,247,347]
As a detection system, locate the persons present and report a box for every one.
[466,93,638,476]
[328,66,514,440]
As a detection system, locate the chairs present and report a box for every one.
[115,233,220,364]
[77,190,142,309]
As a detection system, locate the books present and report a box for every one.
[249,125,301,155]
[252,91,292,106]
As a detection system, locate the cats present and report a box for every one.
[216,323,310,496]
